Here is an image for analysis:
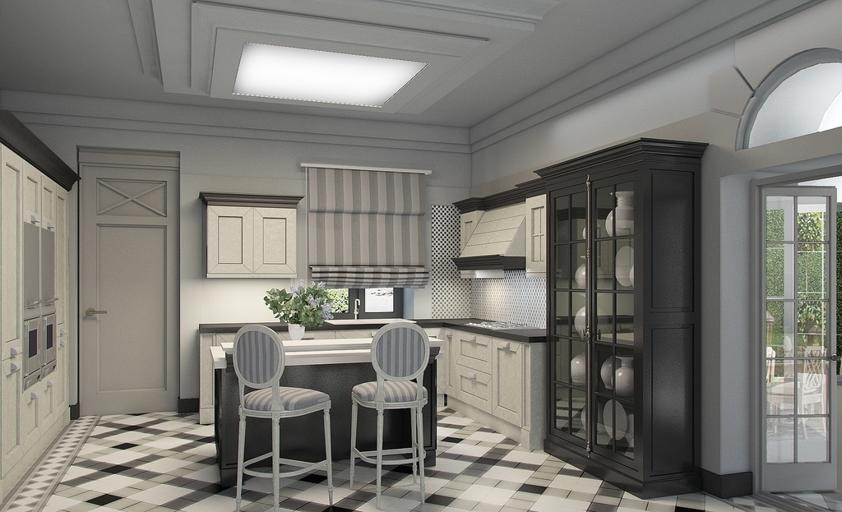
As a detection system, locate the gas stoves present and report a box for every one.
[465,319,536,331]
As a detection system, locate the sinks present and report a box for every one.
[323,318,417,326]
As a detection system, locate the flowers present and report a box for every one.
[263,278,337,326]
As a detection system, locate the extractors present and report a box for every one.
[451,205,525,271]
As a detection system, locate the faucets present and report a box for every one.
[354,299,360,320]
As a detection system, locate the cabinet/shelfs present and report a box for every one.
[199,328,445,424]
[446,323,493,449]
[198,192,304,278]
[0,105,82,512]
[494,330,547,452]
[534,137,709,500]
[515,178,546,277]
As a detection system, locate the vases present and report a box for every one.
[571,191,634,432]
[287,323,305,341]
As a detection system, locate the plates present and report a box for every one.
[603,400,628,440]
[615,245,635,286]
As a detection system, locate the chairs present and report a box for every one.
[350,321,431,510]
[232,325,334,512]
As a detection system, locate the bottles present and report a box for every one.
[573,293,586,335]
[574,256,586,288]
[606,191,634,235]
[571,350,634,399]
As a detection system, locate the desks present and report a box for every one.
[209,338,446,469]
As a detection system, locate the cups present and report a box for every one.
[288,324,305,340]
